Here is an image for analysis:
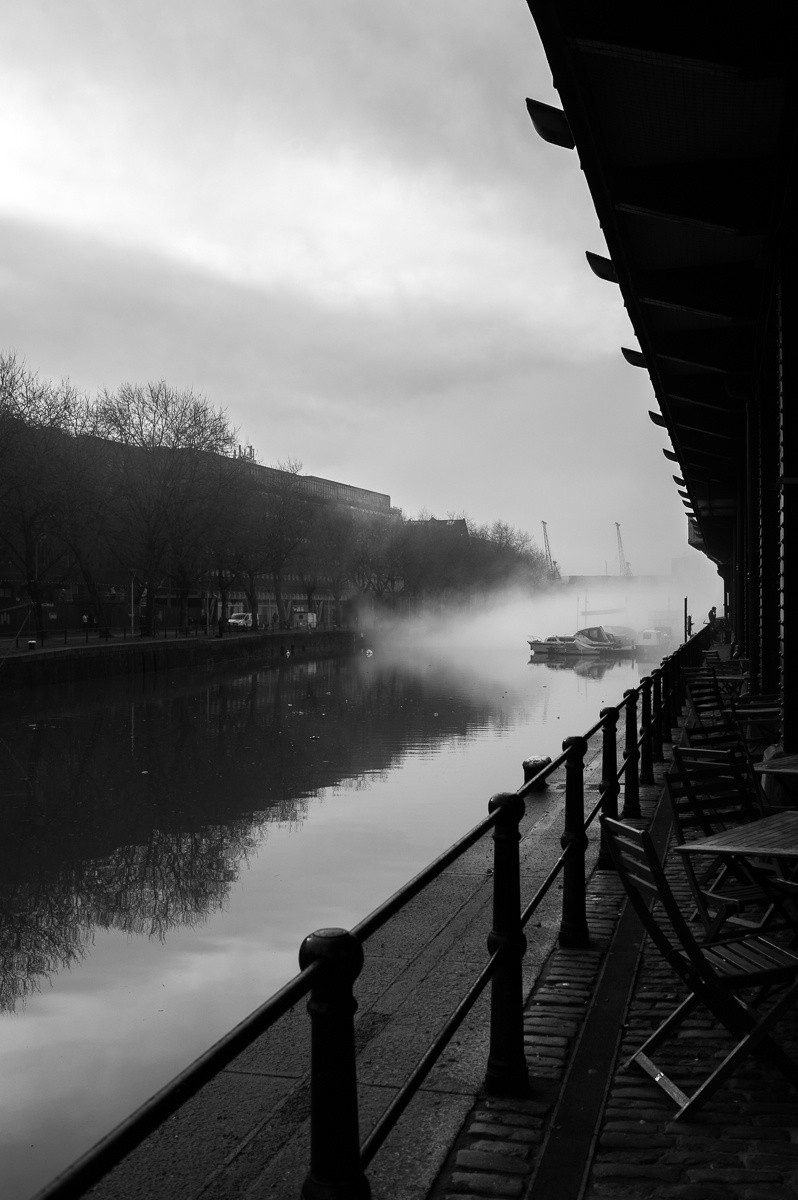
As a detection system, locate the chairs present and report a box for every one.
[598,650,798,1121]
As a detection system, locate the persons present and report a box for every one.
[272,613,278,627]
[708,607,716,622]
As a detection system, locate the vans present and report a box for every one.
[227,613,259,631]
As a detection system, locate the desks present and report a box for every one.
[721,708,783,746]
[676,811,798,955]
[753,754,798,811]
[704,676,751,696]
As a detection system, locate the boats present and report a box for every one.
[525,625,673,656]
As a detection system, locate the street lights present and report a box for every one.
[35,536,50,644]
[126,568,138,641]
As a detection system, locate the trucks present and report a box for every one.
[293,612,326,631]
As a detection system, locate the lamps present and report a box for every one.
[649,411,666,427]
[663,449,678,462]
[586,250,619,283]
[621,347,647,368]
[526,98,575,149]
[673,476,696,518]
